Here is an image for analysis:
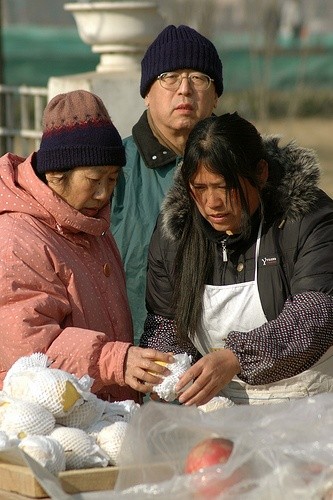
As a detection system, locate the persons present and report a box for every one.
[136,111,333,407]
[102,24,224,406]
[0,90,176,407]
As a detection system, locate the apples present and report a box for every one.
[187,437,250,499]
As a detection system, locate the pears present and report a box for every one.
[0,351,240,467]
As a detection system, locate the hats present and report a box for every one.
[35,90,127,173]
[140,24,224,98]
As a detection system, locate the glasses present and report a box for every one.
[156,72,214,90]
